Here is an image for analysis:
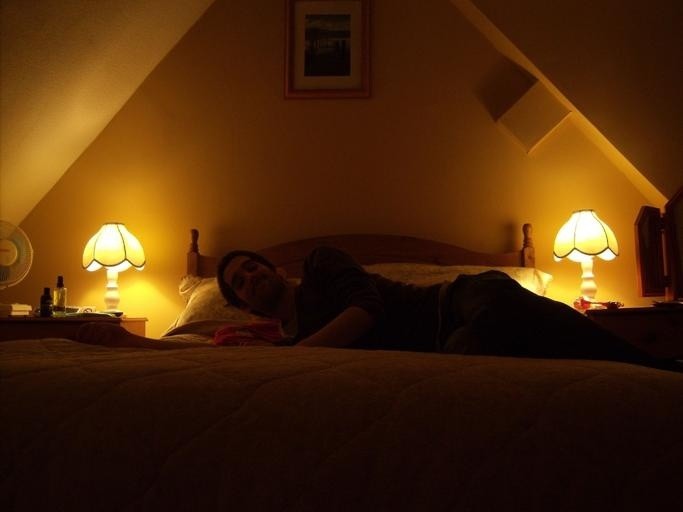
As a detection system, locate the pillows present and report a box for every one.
[172,264,554,346]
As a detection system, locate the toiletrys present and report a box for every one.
[39,286,53,319]
[51,274,67,317]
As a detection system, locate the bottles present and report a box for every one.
[40,276,67,318]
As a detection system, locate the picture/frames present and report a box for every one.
[284,0,374,101]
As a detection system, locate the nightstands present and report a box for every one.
[584,304,666,359]
[0,315,147,340]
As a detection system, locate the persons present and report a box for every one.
[71,244,682,374]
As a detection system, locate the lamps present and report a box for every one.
[82,221,146,318]
[553,208,620,308]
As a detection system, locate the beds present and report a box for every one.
[0,223,682,511]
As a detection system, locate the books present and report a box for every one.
[0,303,33,312]
[0,310,30,318]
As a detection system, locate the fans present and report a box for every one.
[0,220,34,288]
